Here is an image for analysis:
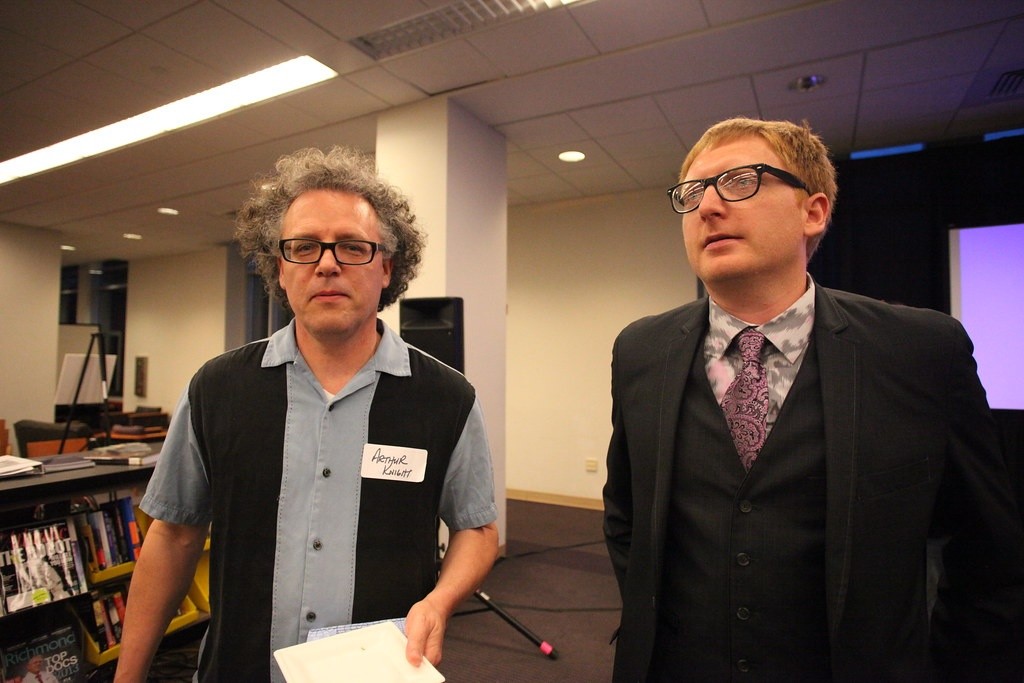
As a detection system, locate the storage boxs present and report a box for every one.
[0,507,214,683]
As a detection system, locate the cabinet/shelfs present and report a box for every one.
[0,442,213,682]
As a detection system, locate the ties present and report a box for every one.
[719,327,769,475]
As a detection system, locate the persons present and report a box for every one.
[22,654,59,683]
[601,118,1024,683]
[115,146,498,683]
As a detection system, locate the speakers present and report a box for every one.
[398,296,465,375]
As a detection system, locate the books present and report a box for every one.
[87,442,165,466]
[0,454,142,683]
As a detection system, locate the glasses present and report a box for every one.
[277,238,386,265]
[667,163,811,215]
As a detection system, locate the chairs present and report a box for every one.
[16,400,170,460]
